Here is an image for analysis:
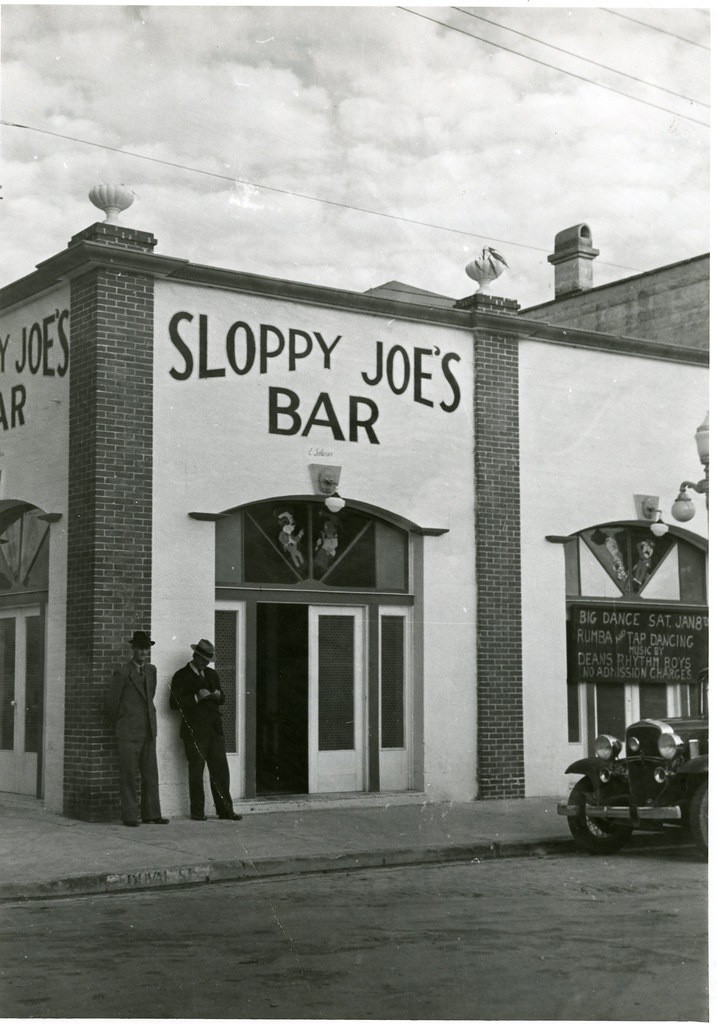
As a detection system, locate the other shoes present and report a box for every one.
[123,821,141,827]
[192,816,208,820]
[142,817,170,824]
[219,813,242,820]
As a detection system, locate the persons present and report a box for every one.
[170,638,242,820]
[107,630,169,826]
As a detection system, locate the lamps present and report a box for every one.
[634,494,669,536]
[308,464,345,512]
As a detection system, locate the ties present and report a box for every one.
[198,670,203,677]
[140,666,144,675]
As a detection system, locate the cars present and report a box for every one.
[564,669,708,859]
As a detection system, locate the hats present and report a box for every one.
[128,631,155,647]
[190,639,218,662]
